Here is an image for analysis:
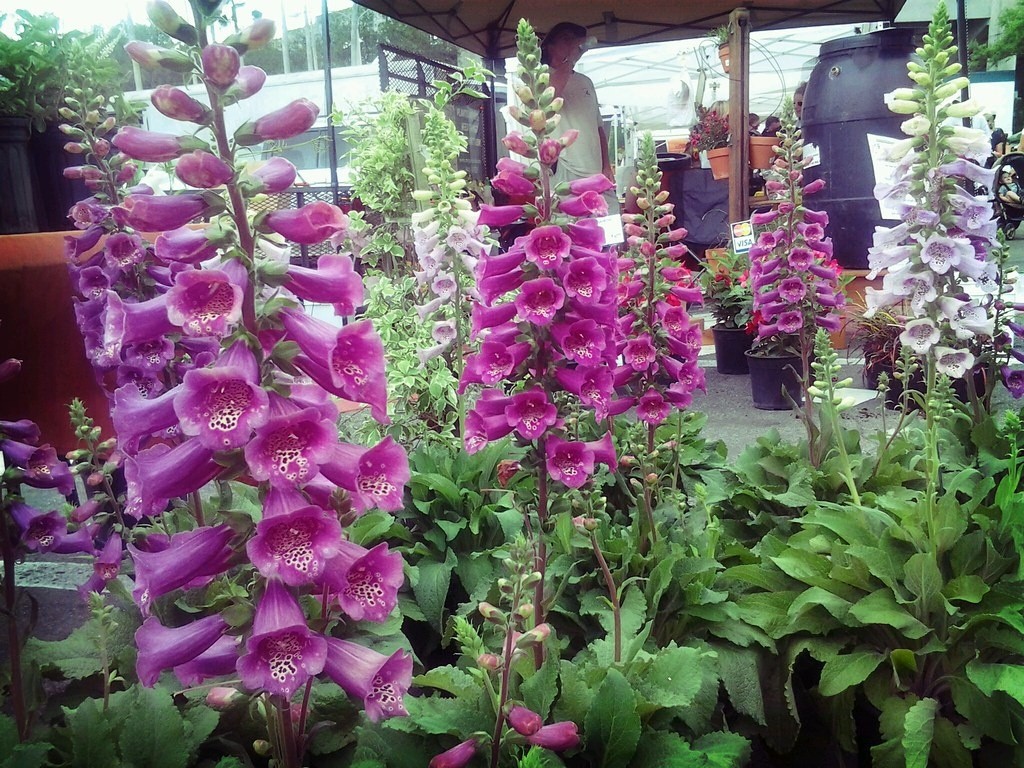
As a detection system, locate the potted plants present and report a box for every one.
[0,8,150,234]
[840,290,909,389]
[708,26,731,73]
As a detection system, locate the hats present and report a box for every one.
[540,22,586,65]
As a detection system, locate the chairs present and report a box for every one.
[667,166,731,289]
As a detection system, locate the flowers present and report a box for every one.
[0,0,1024,768]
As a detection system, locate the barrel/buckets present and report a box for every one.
[632,149,692,247]
[0,224,215,455]
[801,33,926,270]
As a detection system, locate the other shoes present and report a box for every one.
[1020,197,1024,204]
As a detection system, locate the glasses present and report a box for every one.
[794,102,802,106]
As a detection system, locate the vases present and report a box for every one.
[710,325,754,374]
[750,135,782,169]
[744,346,804,410]
[952,368,995,412]
[880,364,926,412]
[706,146,730,181]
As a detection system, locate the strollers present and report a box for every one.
[985,150,1024,240]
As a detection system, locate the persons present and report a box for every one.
[538,23,622,221]
[749,82,808,138]
[983,112,1024,205]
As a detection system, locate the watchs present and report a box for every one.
[561,57,576,69]
